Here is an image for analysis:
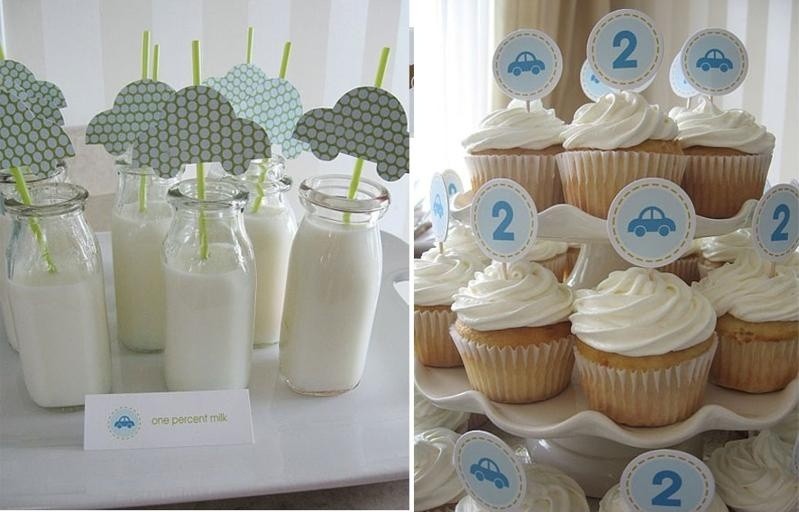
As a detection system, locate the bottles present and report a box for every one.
[2,167,84,353]
[241,177,297,346]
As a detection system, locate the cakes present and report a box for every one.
[440,193,574,265]
[451,260,575,402]
[415,388,471,432]
[571,267,718,428]
[670,98,775,216]
[414,249,482,367]
[557,91,687,221]
[702,231,798,393]
[460,97,566,214]
[602,475,727,512]
[714,431,799,512]
[454,462,590,512]
[410,426,474,511]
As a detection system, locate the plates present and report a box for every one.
[414,356,799,457]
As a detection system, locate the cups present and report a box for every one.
[211,162,302,350]
